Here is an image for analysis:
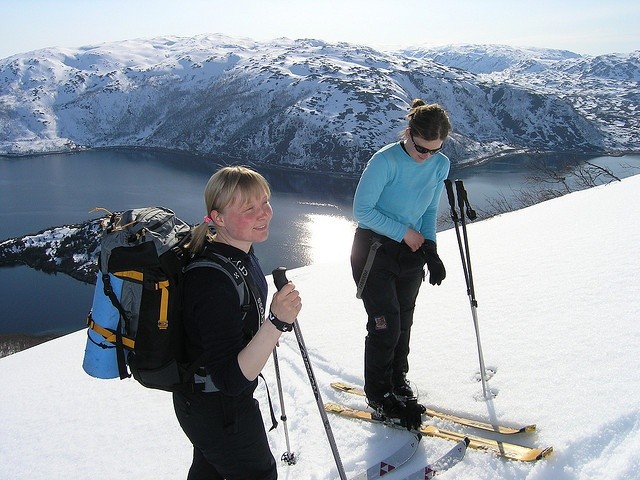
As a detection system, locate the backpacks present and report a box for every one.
[81,206,251,393]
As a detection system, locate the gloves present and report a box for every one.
[422,240,446,285]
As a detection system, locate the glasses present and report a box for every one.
[410,133,444,155]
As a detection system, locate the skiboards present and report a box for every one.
[322,382,553,461]
[350,433,470,480]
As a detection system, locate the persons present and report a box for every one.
[349,98,451,429]
[172,165,302,480]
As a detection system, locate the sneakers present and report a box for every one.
[365,389,405,424]
[390,380,413,399]
